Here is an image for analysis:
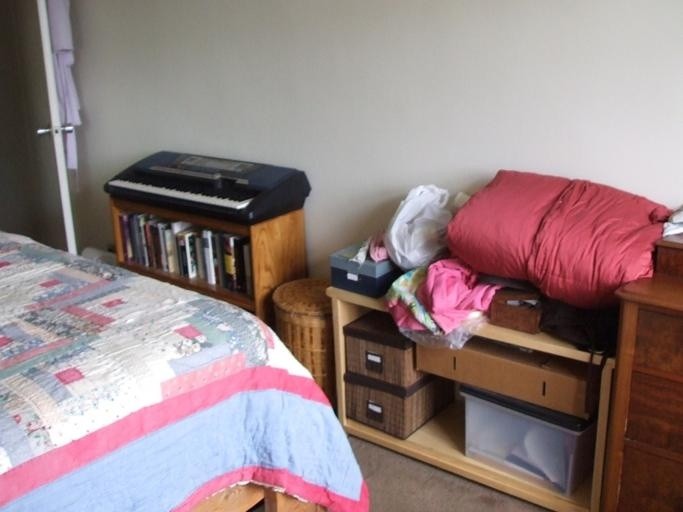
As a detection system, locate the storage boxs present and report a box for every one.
[328,239,404,300]
[343,309,437,441]
[458,382,596,497]
[490,292,543,334]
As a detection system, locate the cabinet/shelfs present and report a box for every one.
[614,236,681,512]
[108,195,308,331]
[325,285,616,512]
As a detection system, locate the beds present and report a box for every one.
[0,230,328,512]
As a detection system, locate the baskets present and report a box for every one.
[343,372,456,440]
[343,309,432,388]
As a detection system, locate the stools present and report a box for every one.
[272,277,336,407]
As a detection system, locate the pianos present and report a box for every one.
[104,152,310,226]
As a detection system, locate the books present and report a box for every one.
[117,211,253,299]
